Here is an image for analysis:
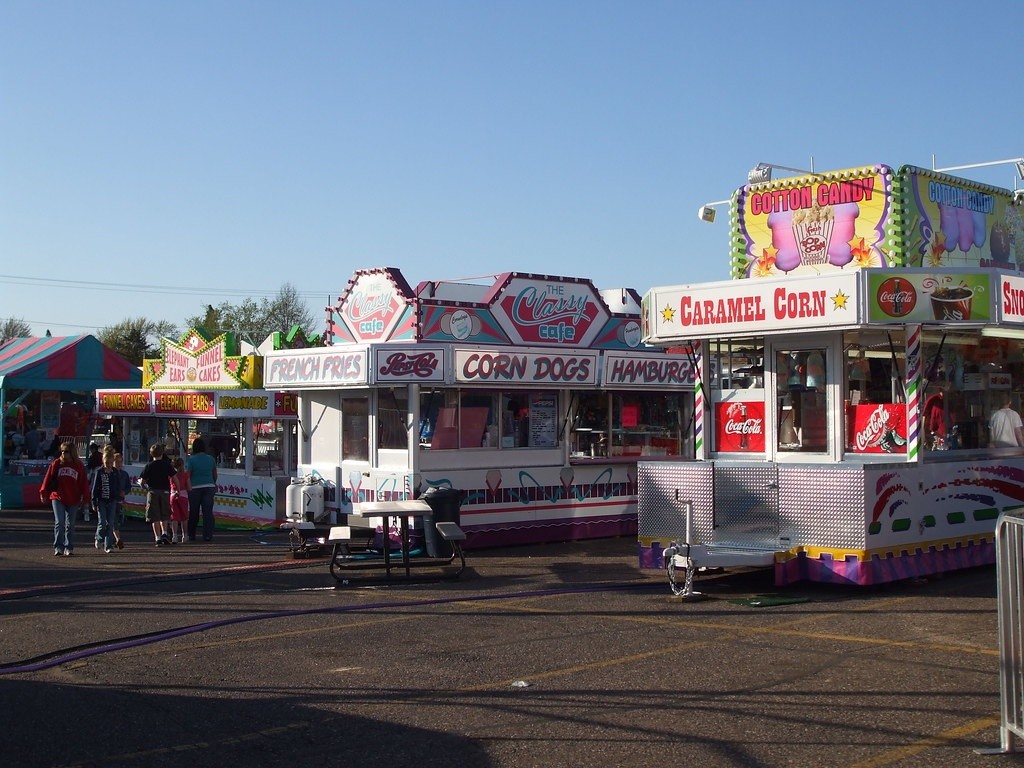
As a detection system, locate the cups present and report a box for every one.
[577,452,585,460]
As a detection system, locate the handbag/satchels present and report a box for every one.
[47,460,60,490]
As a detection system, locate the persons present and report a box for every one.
[988,392,1024,447]
[140,443,180,547]
[923,381,964,449]
[186,438,217,541]
[90,444,103,468]
[164,433,174,454]
[39,441,90,556]
[91,445,122,553]
[209,436,240,468]
[170,457,192,544]
[108,432,122,454]
[24,423,49,459]
[113,453,132,549]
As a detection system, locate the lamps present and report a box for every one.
[698,199,731,222]
[748,162,817,183]
[934,158,1024,180]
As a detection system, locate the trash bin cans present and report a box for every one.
[418,488,466,558]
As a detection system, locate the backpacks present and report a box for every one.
[4,433,17,455]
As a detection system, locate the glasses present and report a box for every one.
[61,449,70,453]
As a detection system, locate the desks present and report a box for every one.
[360,500,432,586]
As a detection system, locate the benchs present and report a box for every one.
[328,526,351,586]
[436,522,467,578]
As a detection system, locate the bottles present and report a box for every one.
[894,279,903,313]
[590,443,595,459]
[84,508,90,521]
[739,405,749,448]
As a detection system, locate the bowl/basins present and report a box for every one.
[930,286,975,321]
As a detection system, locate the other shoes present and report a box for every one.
[172,536,178,544]
[204,534,211,541]
[161,533,171,545]
[181,536,189,543]
[95,538,102,549]
[55,547,63,556]
[105,546,111,553]
[115,539,124,549]
[64,547,73,556]
[155,539,163,546]
[189,535,197,542]
